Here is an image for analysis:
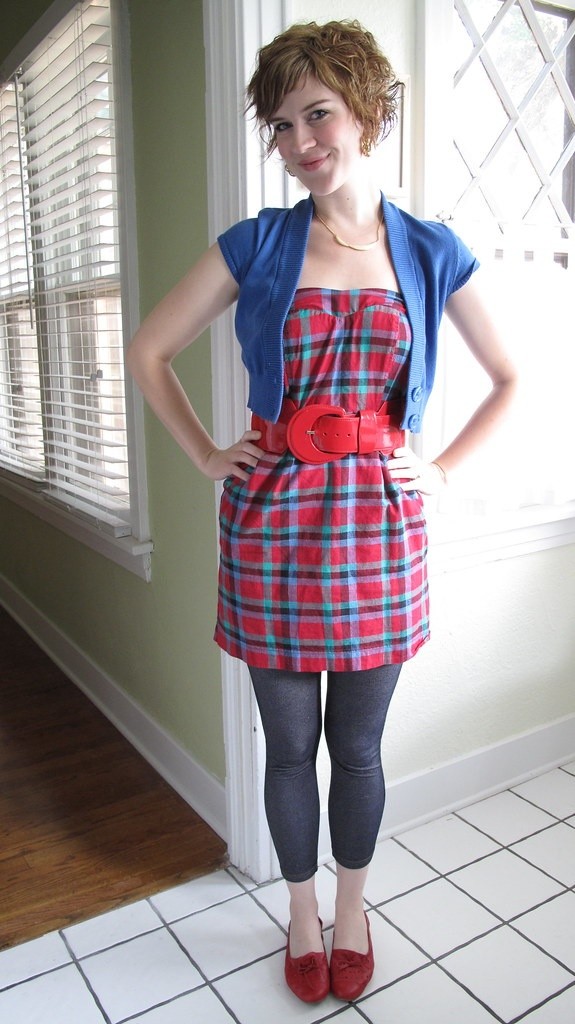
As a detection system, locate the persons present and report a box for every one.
[123,18,521,1002]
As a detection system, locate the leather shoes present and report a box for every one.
[285,916,330,1004]
[330,910,374,1001]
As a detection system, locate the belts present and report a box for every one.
[250,397,405,466]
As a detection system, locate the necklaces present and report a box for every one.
[313,211,384,251]
